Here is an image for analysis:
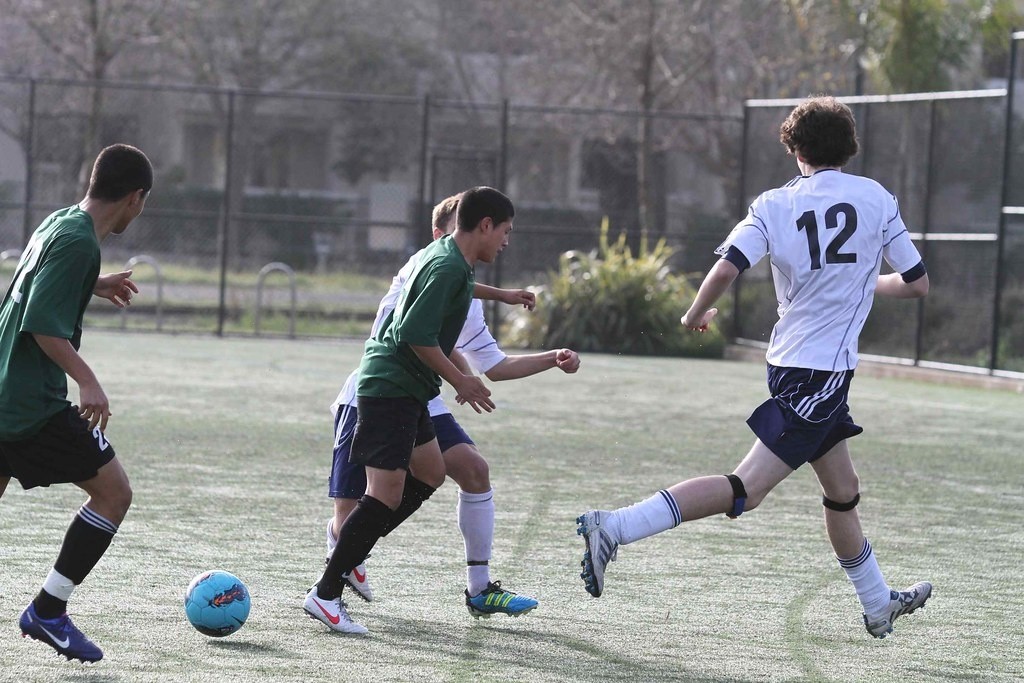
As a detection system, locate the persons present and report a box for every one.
[303,184,514,635]
[328,192,580,617]
[576,92,933,638]
[0,144,153,665]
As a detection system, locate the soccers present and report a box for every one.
[184,570,250,637]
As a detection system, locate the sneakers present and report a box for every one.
[863,580,934,639]
[302,588,369,634]
[327,545,373,603]
[17,601,105,663]
[575,506,619,598]
[464,581,537,620]
